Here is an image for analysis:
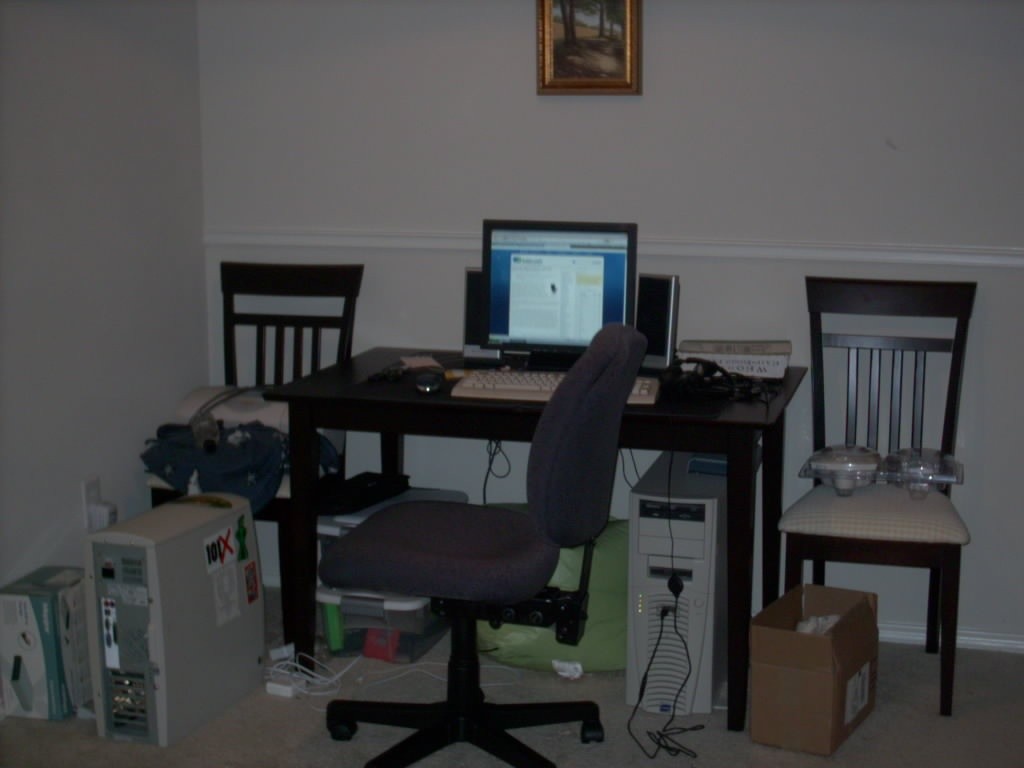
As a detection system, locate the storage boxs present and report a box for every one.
[316,489,470,664]
[0,566,92,721]
[750,585,879,753]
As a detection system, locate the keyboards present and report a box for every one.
[450,370,660,405]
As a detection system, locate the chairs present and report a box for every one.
[320,325,650,768]
[778,276,977,715]
[146,262,365,682]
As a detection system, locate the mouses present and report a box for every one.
[416,375,441,393]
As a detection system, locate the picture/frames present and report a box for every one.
[536,0,641,95]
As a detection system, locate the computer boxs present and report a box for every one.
[627,450,734,715]
[85,494,268,745]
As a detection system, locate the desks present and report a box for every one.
[263,347,807,733]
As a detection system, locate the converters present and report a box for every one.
[266,673,307,698]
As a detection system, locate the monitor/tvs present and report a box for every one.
[481,219,639,374]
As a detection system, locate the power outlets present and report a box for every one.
[83,476,100,531]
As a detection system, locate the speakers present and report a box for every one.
[462,268,503,359]
[636,274,680,369]
